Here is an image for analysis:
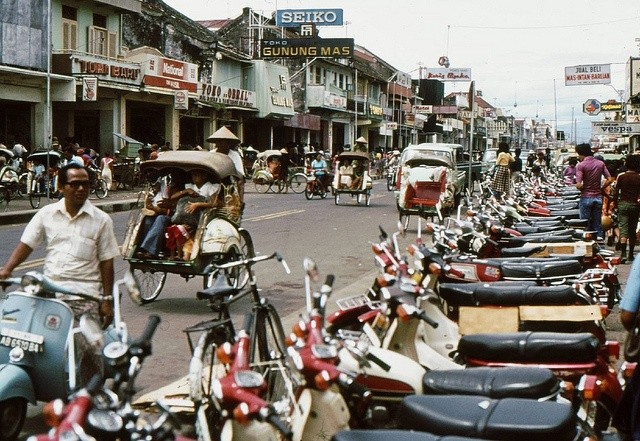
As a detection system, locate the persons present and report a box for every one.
[526,149,550,168]
[610,250,639,441]
[375,148,402,191]
[138,141,202,161]
[206,125,245,211]
[490,142,522,200]
[47,134,119,200]
[136,171,217,266]
[348,159,365,190]
[288,142,340,197]
[30,160,46,193]
[340,158,353,189]
[279,149,288,194]
[574,143,612,241]
[235,142,259,158]
[564,157,577,183]
[604,154,640,261]
[1,164,121,386]
[352,136,368,152]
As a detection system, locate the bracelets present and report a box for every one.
[103,294,114,301]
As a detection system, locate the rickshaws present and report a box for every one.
[251,149,308,196]
[397,150,458,234]
[0,137,150,208]
[335,151,372,207]
[122,149,256,313]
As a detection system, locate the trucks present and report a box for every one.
[396,141,467,202]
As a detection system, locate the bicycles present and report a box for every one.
[182,251,287,415]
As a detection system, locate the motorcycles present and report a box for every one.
[404,202,610,303]
[464,146,591,201]
[352,243,625,432]
[409,226,607,254]
[304,167,329,201]
[26,315,204,441]
[387,165,396,192]
[0,271,128,441]
[186,310,601,441]
[326,225,609,338]
[427,202,588,224]
[284,257,620,434]
[411,254,608,285]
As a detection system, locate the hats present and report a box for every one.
[206,125,241,144]
[355,137,367,144]
[281,149,288,153]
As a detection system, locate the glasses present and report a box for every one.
[65,180,89,188]
